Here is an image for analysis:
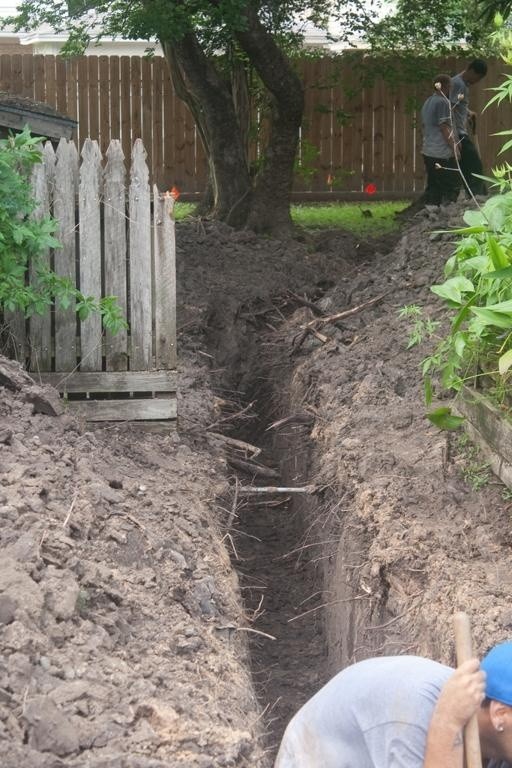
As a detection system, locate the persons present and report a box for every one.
[272,640,511,768]
[419,72,461,208]
[449,58,488,200]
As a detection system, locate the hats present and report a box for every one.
[479,641,512,706]
